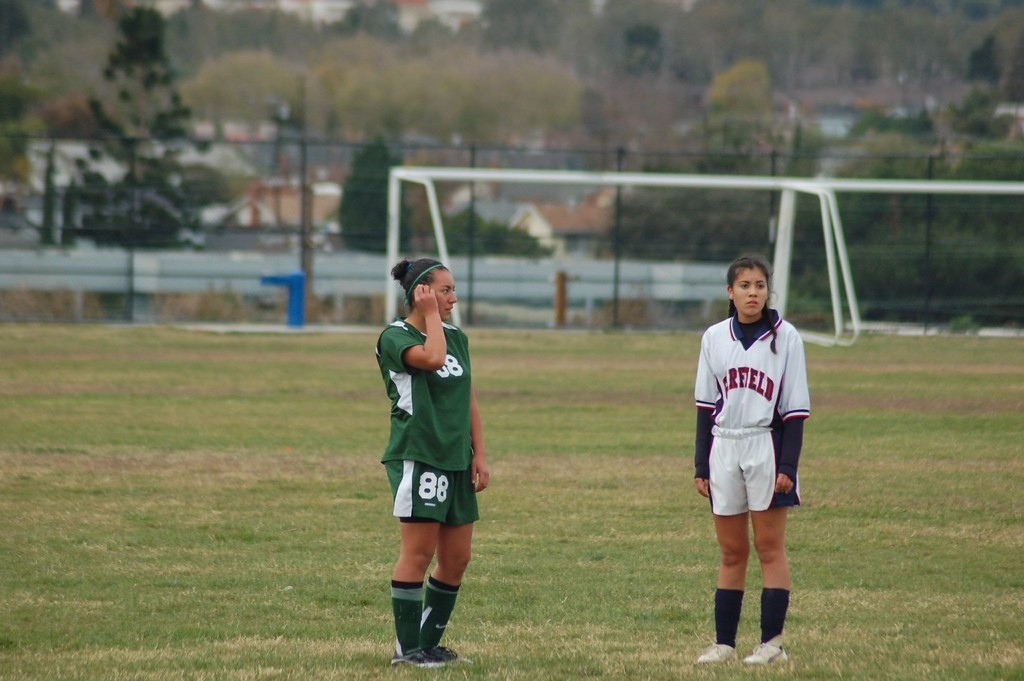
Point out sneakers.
[390,649,446,668]
[696,642,737,665]
[743,644,787,665]
[420,645,475,667]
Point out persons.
[694,256,810,665]
[376,258,489,670]
